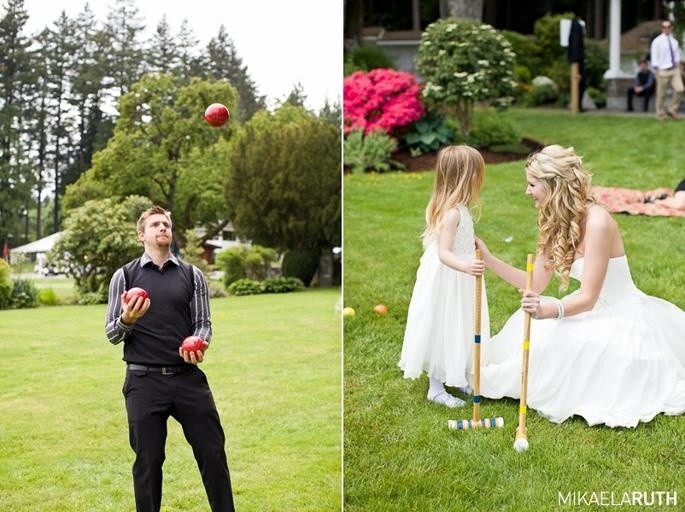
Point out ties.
[668,35,676,68]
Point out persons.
[644,180,685,209]
[627,61,655,113]
[399,144,491,408]
[475,144,684,429]
[104,206,235,512]
[650,20,685,119]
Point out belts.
[128,365,183,376]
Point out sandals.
[427,390,466,408]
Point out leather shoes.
[657,109,679,122]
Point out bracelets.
[555,300,565,320]
